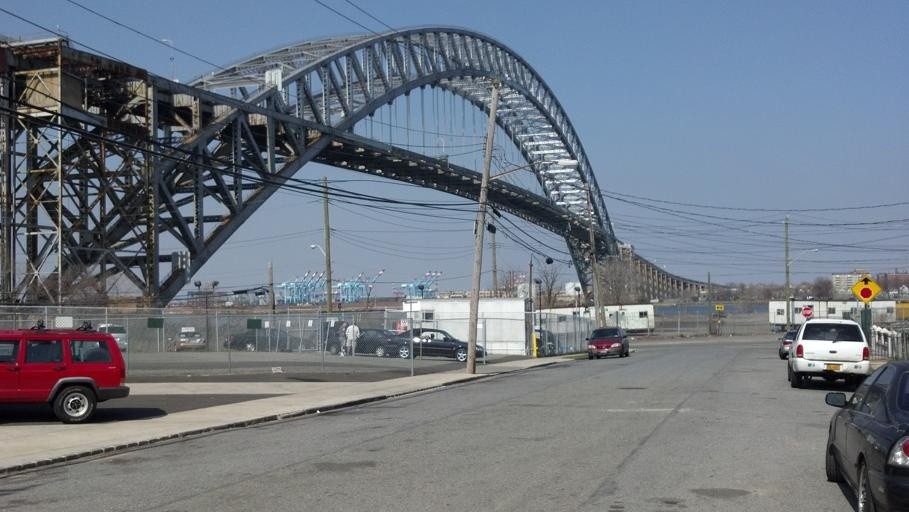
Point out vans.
[97,323,128,351]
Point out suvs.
[0,320,129,424]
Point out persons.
[337,322,348,355]
[344,323,361,356]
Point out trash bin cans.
[710,312,720,333]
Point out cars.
[778,330,798,359]
[825,360,909,512]
[388,328,487,362]
[788,317,870,387]
[586,327,629,357]
[166,331,205,351]
[224,327,313,351]
[534,329,560,357]
[325,328,395,357]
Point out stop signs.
[801,308,812,317]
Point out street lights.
[782,248,818,329]
[465,158,580,372]
[213,281,220,304]
[194,281,201,293]
[418,285,423,360]
[533,277,548,327]
[310,244,333,312]
